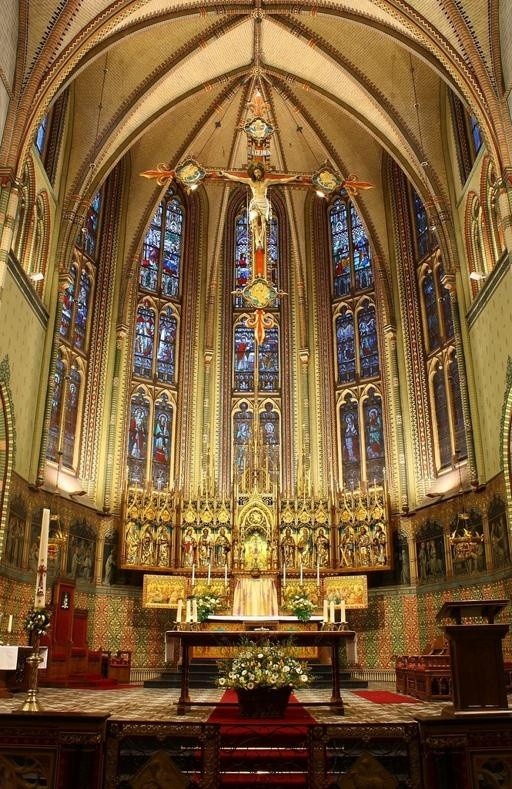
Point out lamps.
[425,492,446,498]
[69,490,87,496]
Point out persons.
[125,510,387,566]
[398,517,507,588]
[133,405,379,465]
[8,514,116,586]
[217,164,299,253]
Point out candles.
[176,563,227,623]
[317,563,319,586]
[300,564,302,585]
[283,563,285,586]
[323,599,346,623]
[7,614,13,633]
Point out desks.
[0,645,48,698]
[166,630,355,716]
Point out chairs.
[390,636,454,702]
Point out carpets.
[349,689,424,704]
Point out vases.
[234,685,292,719]
[18,630,46,712]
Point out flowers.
[187,592,225,622]
[285,592,316,623]
[207,631,317,692]
[22,608,54,636]
